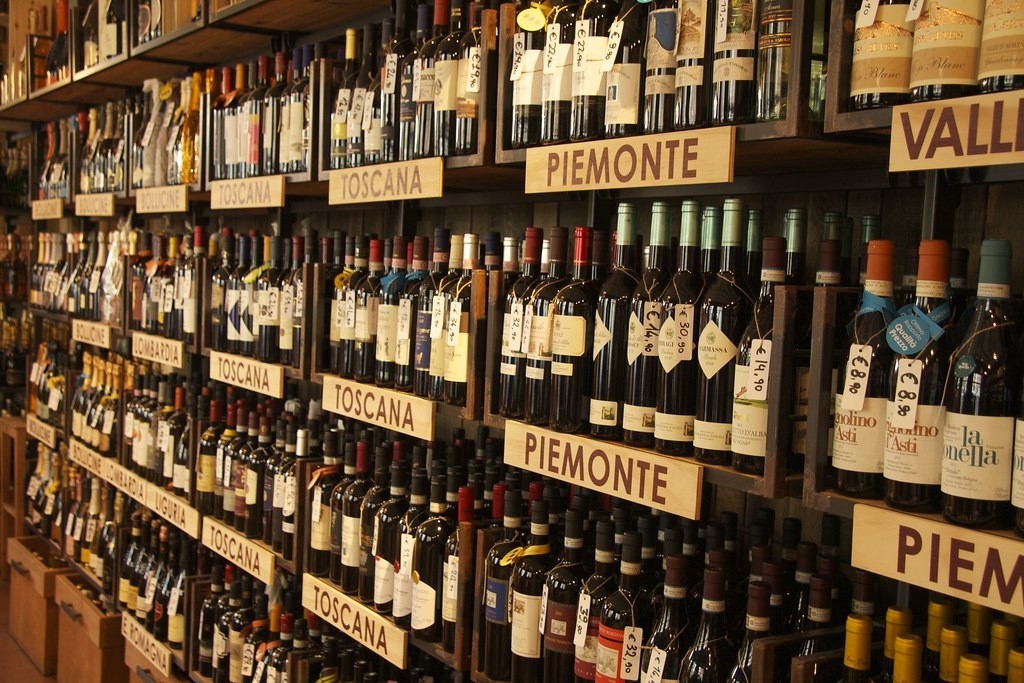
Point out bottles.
[0,0,1024,683]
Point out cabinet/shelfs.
[0,0,1023,683]
